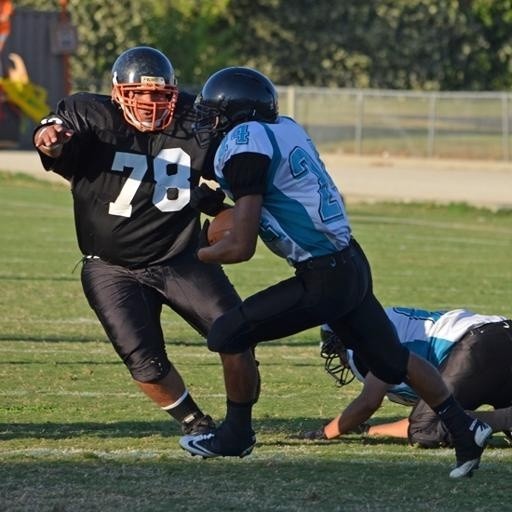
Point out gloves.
[341,423,371,437]
[190,181,226,217]
[192,218,210,265]
[300,424,327,440]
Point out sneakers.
[179,421,256,459]
[449,418,493,479]
[180,412,217,437]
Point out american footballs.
[208,210,234,246]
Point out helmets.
[319,321,357,387]
[195,66,280,126]
[110,44,176,103]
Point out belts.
[72,253,100,273]
[468,318,511,335]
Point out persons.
[321,307,511,448]
[34,46,242,435]
[179,67,494,478]
